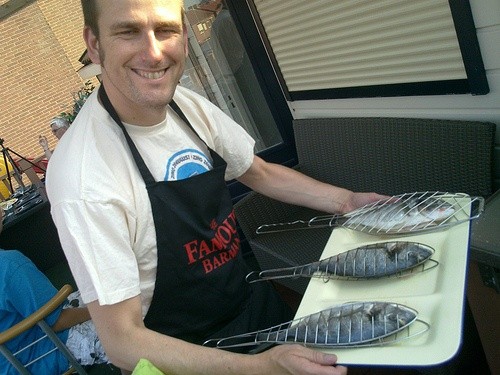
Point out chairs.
[0,284,88,375]
[233,119,496,295]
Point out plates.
[290,193,472,366]
[13,184,42,214]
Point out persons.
[39,0,491,375]
[0,203,120,375]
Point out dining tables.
[0,179,49,231]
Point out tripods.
[0,139,46,196]
